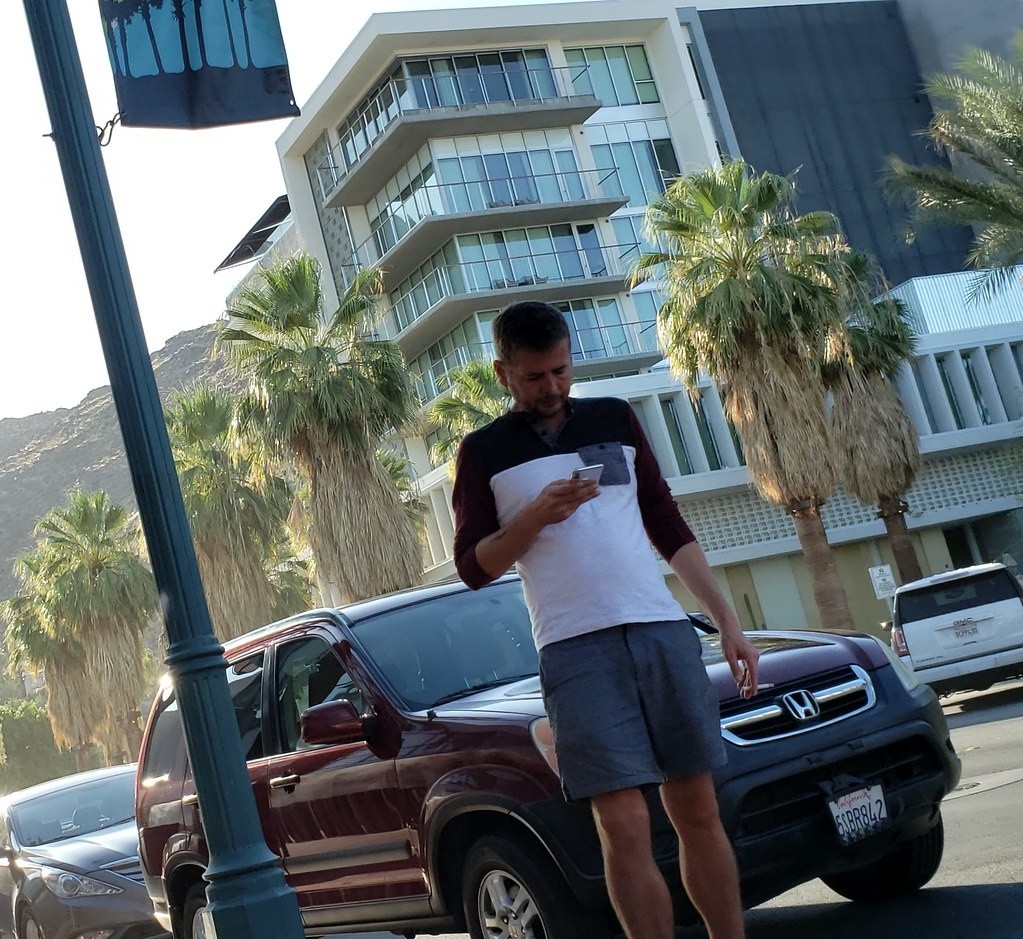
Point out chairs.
[523,273,549,285]
[307,642,423,713]
[71,806,103,830]
[449,623,511,689]
[487,197,540,208]
[493,278,521,287]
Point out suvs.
[133,573,964,939]
[881,561,1023,700]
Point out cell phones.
[571,463,604,490]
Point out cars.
[0,762,174,939]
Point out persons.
[452,301,759,939]
